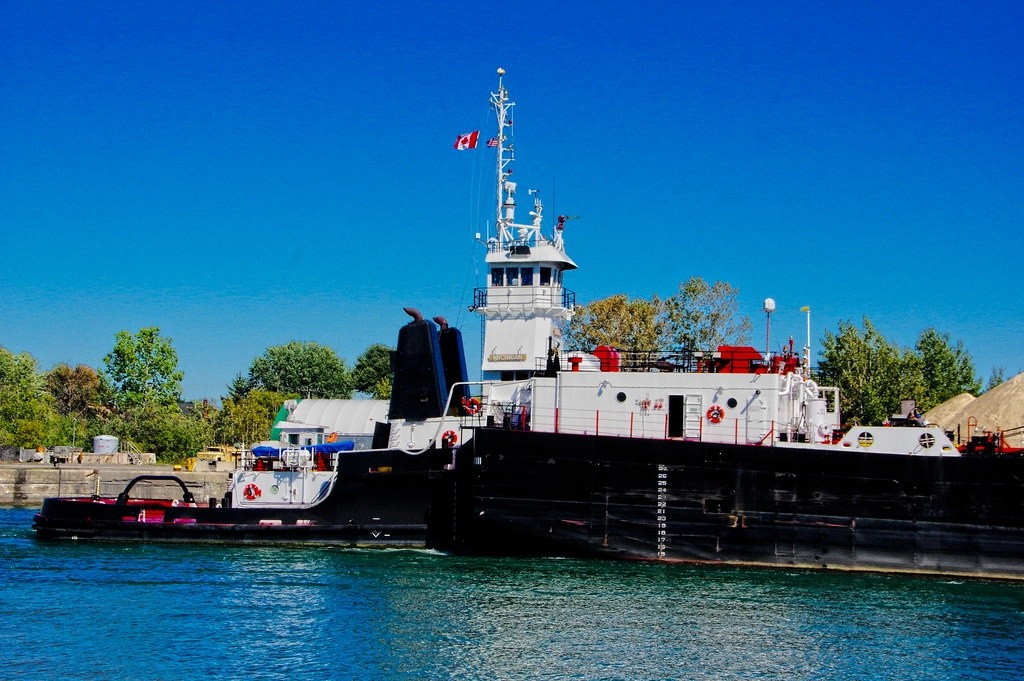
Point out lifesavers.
[707,406,724,423]
[442,430,457,446]
[465,398,481,414]
[243,484,259,500]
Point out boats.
[31,68,1023,581]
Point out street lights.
[801,306,810,375]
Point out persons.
[905,406,922,427]
[77,454,83,463]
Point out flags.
[487,138,498,147]
[454,131,478,150]
